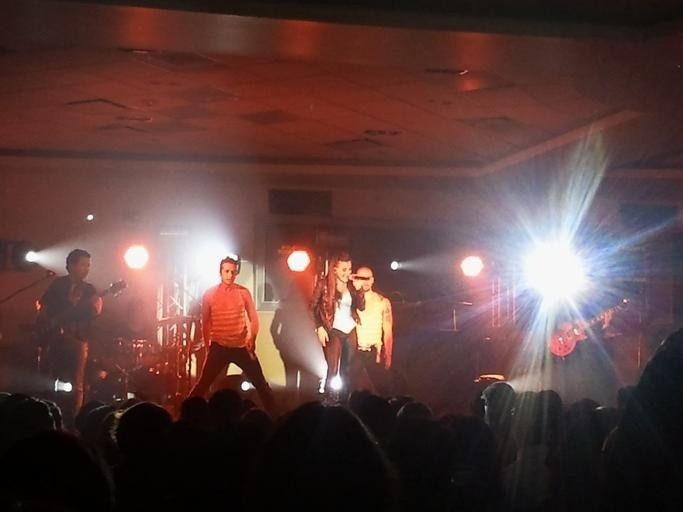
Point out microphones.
[348,275,370,282]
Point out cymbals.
[157,316,201,327]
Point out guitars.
[32,278,129,354]
[544,296,630,357]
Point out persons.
[31,248,104,432]
[354,267,393,396]
[0,327,681,510]
[184,255,280,415]
[302,252,366,403]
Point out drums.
[112,336,153,377]
